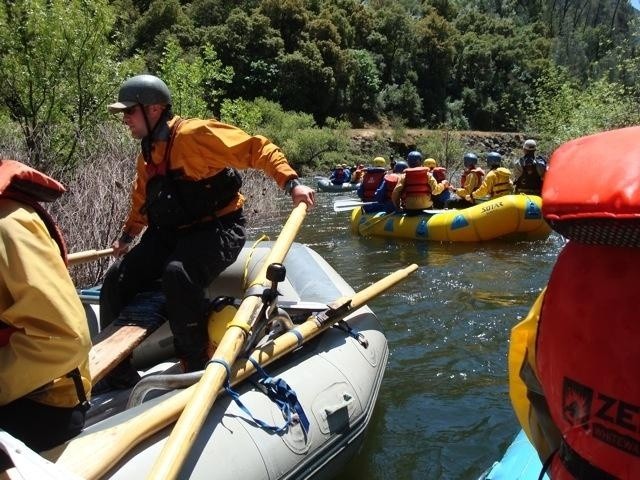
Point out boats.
[479,425,552,480]
[317,178,361,192]
[352,194,553,243]
[1,234,393,477]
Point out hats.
[523,140,537,150]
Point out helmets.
[336,164,364,169]
[407,151,422,167]
[464,153,477,167]
[423,158,436,168]
[107,75,174,116]
[487,152,501,169]
[373,157,386,167]
[393,162,408,173]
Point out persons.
[0,159,93,473]
[507,127,640,480]
[329,140,545,216]
[91,73,317,399]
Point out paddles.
[1,430,85,480]
[423,210,456,213]
[334,201,378,211]
[359,212,396,236]
[2,264,420,479]
